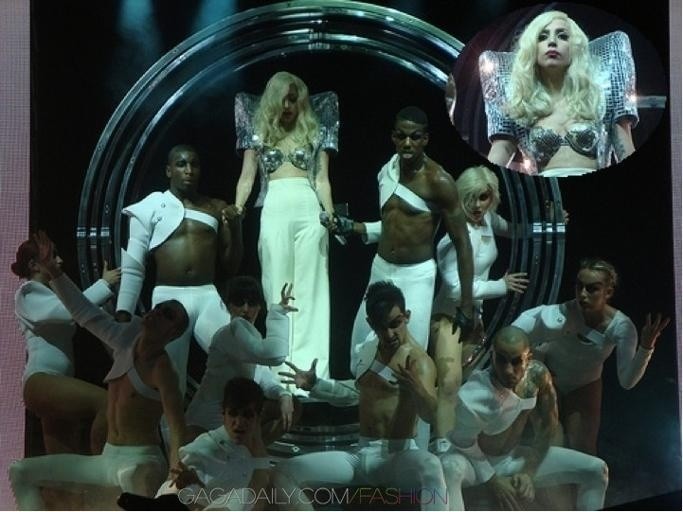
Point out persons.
[325,105,474,374]
[271,280,448,510]
[510,258,672,508]
[8,239,121,457]
[185,277,298,439]
[166,378,313,511]
[476,12,637,178]
[114,141,242,387]
[6,231,182,510]
[431,167,578,431]
[439,325,607,510]
[221,70,343,395]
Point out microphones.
[320,212,349,245]
